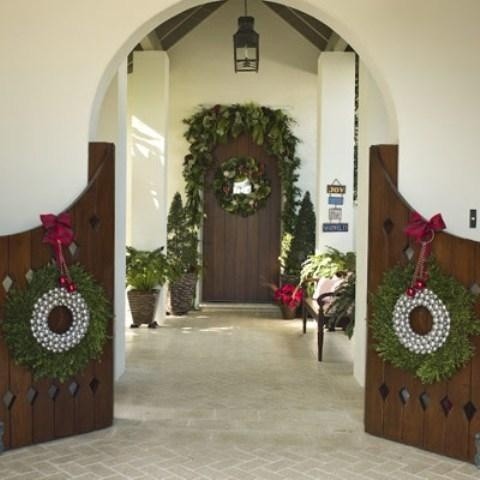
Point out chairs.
[300,270,354,361]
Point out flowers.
[273,284,303,308]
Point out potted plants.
[276,191,315,318]
[166,192,207,315]
[125,245,168,327]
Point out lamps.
[233,1,259,73]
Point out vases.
[279,303,297,318]
